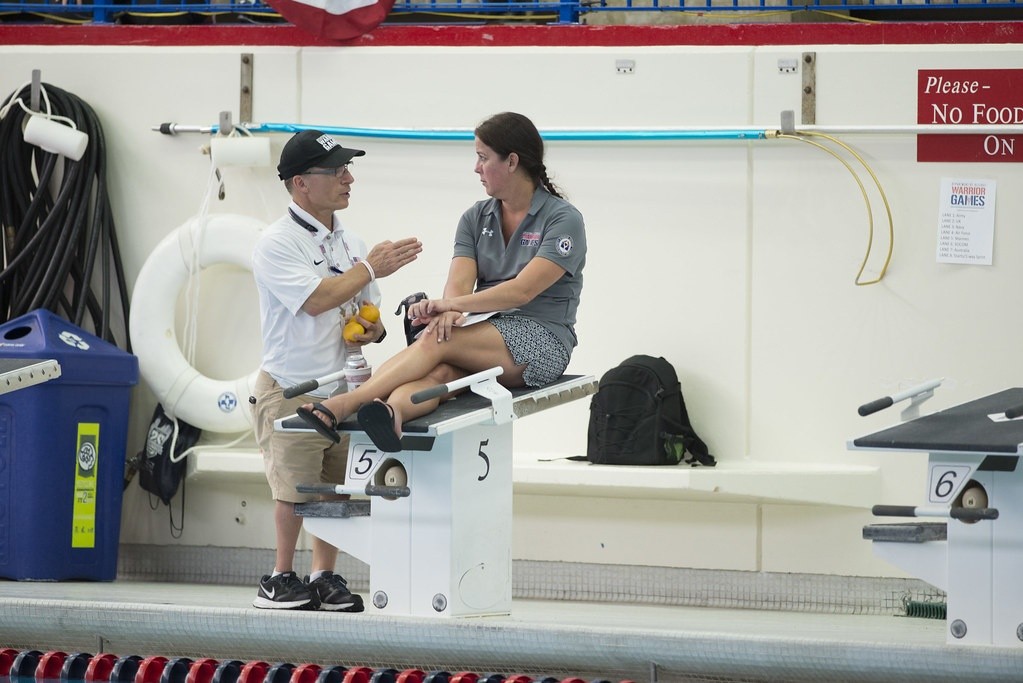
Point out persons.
[253,130,423,612]
[297,112,587,453]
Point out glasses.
[300,161,355,177]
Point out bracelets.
[362,260,375,281]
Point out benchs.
[188,448,878,507]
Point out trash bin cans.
[1,308,140,586]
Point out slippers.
[357,401,402,453]
[296,402,341,443]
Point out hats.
[277,130,366,180]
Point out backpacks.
[137,403,202,539]
[567,354,718,466]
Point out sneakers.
[252,571,319,610]
[303,570,365,612]
